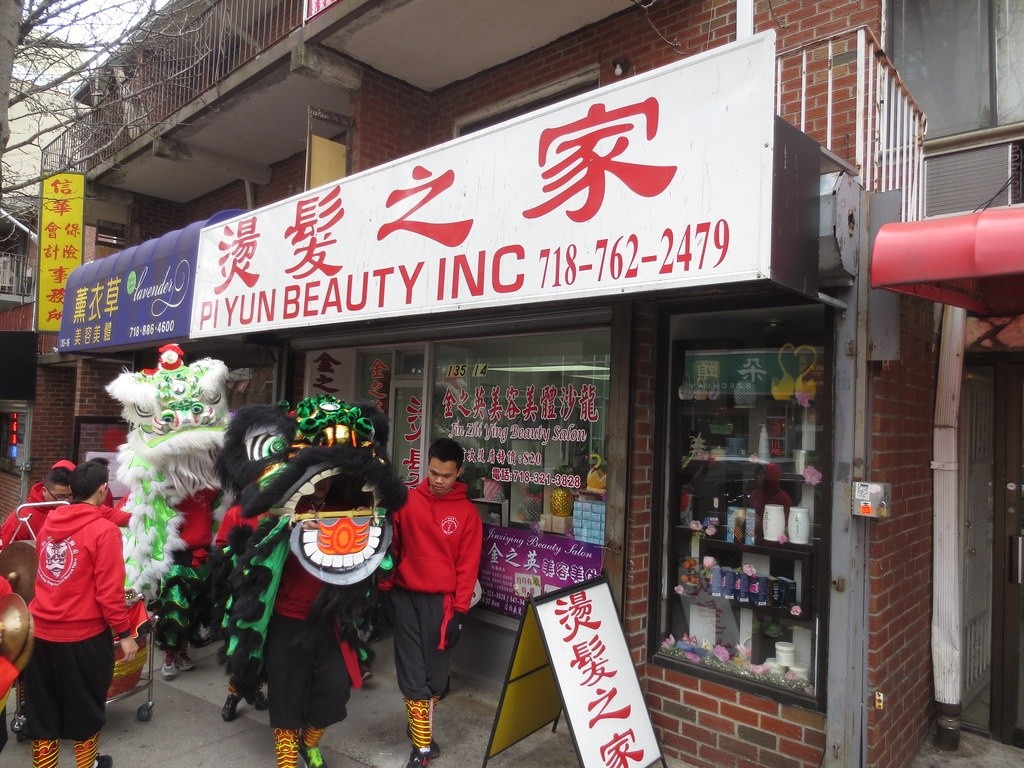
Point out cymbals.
[0,593,34,673]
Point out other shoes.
[369,630,380,642]
[360,662,373,682]
[191,624,215,646]
[95,753,113,768]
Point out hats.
[52,460,76,473]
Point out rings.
[133,654,135,656]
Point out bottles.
[766,642,809,681]
[787,505,810,544]
[763,503,785,542]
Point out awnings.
[57,208,252,352]
[869,207,1024,315]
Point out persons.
[266,473,369,768]
[750,463,792,544]
[378,436,483,768]
[23,463,140,768]
[214,500,266,721]
[0,456,133,753]
[158,488,218,680]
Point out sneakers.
[222,691,243,721]
[160,654,178,681]
[154,638,166,651]
[245,690,267,711]
[297,736,327,768]
[176,652,196,671]
[406,721,440,759]
[406,749,430,768]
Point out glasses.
[44,486,73,502]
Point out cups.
[726,438,748,455]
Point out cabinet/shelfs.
[668,463,820,625]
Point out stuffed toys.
[107,342,407,709]
